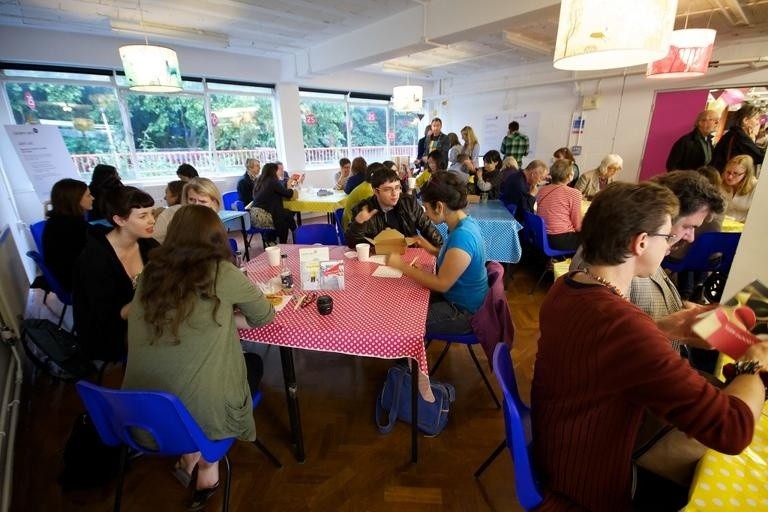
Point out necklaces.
[582,267,631,302]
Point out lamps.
[643,1,716,80]
[118,0,183,92]
[551,1,678,72]
[392,55,423,112]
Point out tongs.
[302,291,317,308]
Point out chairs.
[421,262,504,411]
[507,203,516,216]
[74,378,282,512]
[28,220,51,256]
[222,191,239,210]
[472,341,547,512]
[25,249,74,330]
[522,211,576,295]
[291,223,340,245]
[660,232,740,280]
[336,206,350,244]
[235,201,278,260]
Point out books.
[391,156,411,174]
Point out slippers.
[186,479,220,511]
[170,457,190,488]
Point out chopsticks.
[294,294,306,310]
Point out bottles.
[236,251,247,276]
[281,254,294,295]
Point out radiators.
[0,224,31,343]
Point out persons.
[446,132,462,166]
[534,159,585,250]
[237,159,261,205]
[252,164,297,245]
[696,166,723,187]
[346,156,371,192]
[384,159,413,195]
[417,124,432,162]
[460,124,479,167]
[667,109,721,170]
[498,156,518,183]
[713,106,764,167]
[342,162,384,230]
[573,153,623,201]
[501,120,530,169]
[718,154,757,223]
[553,148,578,186]
[345,170,445,251]
[448,154,471,187]
[501,160,548,220]
[154,177,222,246]
[414,150,446,189]
[86,164,127,219]
[276,160,290,189]
[175,163,200,182]
[334,158,349,188]
[567,170,768,350]
[387,172,492,367]
[75,187,163,373]
[526,181,768,512]
[466,150,503,204]
[124,204,276,506]
[164,181,189,206]
[422,118,448,169]
[42,178,115,300]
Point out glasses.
[648,233,676,241]
[728,171,745,176]
[376,185,400,191]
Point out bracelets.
[721,359,768,401]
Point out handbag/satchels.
[19,319,97,380]
[376,361,456,439]
[250,207,274,230]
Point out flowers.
[395,163,414,184]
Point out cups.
[356,243,370,262]
[480,193,488,204]
[265,246,280,266]
